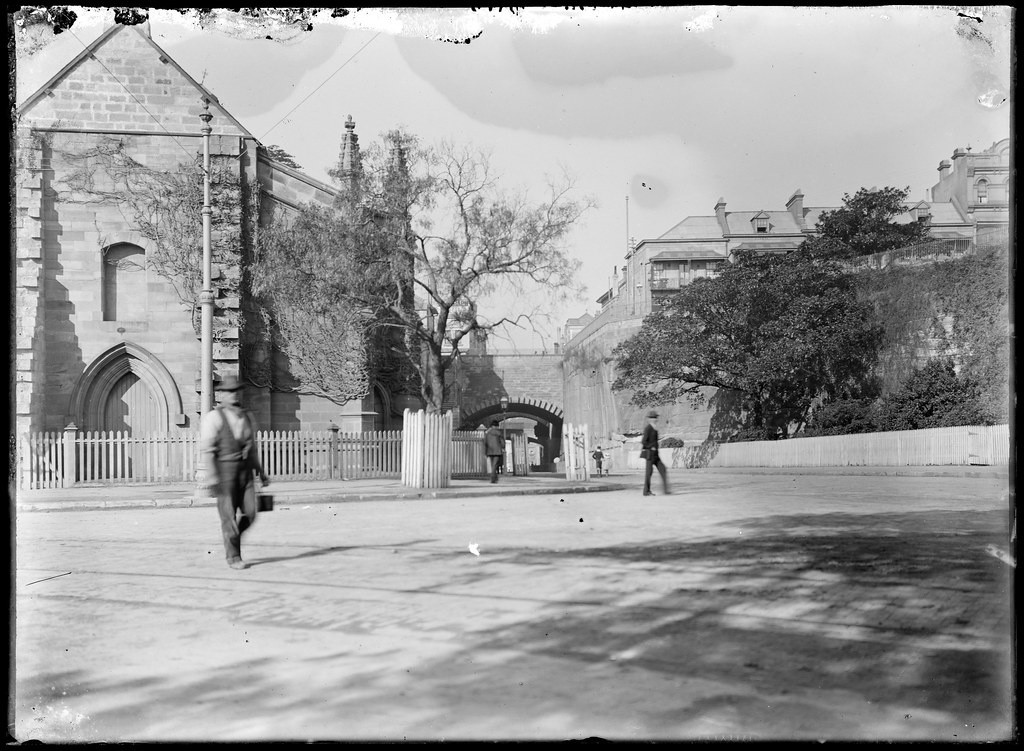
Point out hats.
[214,374,250,392]
[490,420,499,426]
[645,411,660,417]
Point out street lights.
[500,395,508,477]
[196,94,213,498]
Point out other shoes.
[643,493,656,496]
[230,561,247,569]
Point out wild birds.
[468,541,480,556]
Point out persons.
[638,410,672,496]
[603,453,613,477]
[592,447,604,477]
[200,374,271,570]
[484,419,506,483]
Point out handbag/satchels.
[257,485,274,512]
[640,448,649,460]
